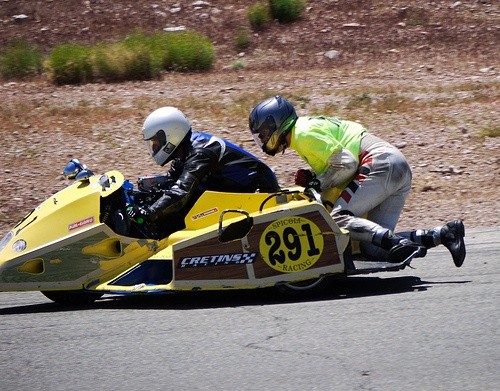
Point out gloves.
[294,169,313,187]
[304,178,321,198]
[128,205,150,219]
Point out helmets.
[140,106,192,166]
[248,95,299,156]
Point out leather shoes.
[439,219,466,267]
[387,230,426,263]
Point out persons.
[128,106,282,225]
[249,96,466,268]
